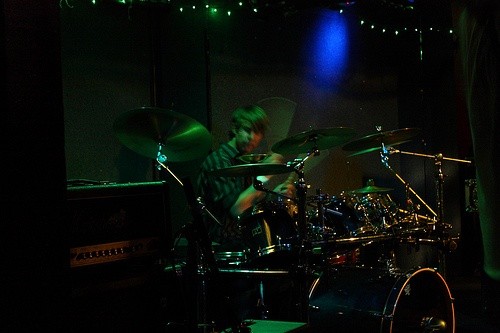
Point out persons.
[197,104,289,250]
[459,0,500,282]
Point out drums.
[255,244,300,274]
[308,266,455,333]
[353,193,399,226]
[213,250,252,271]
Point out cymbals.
[207,162,296,177]
[236,153,266,162]
[342,128,419,152]
[347,186,394,194]
[111,106,211,163]
[345,140,412,159]
[271,127,357,155]
